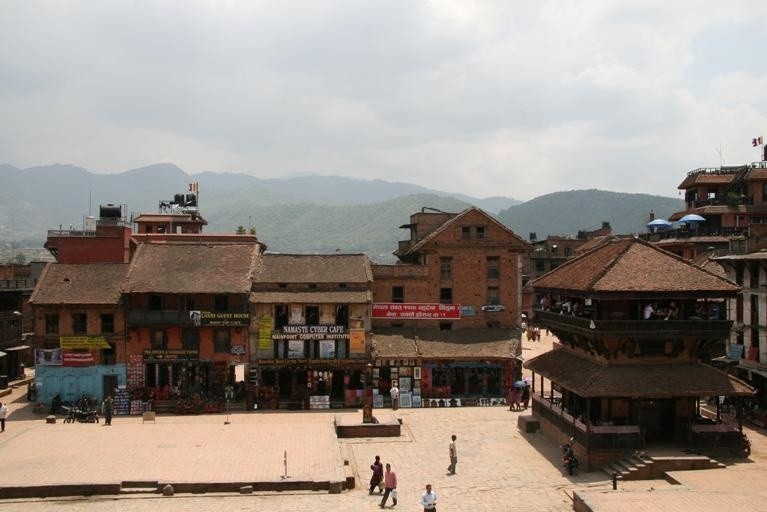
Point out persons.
[421,485,437,512]
[0,402,8,431]
[368,456,383,495]
[447,434,457,474]
[390,384,399,410]
[563,444,572,456]
[509,381,530,410]
[561,448,568,462]
[102,394,114,424]
[378,464,397,508]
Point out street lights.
[14,311,38,367]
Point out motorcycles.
[62,401,101,425]
[559,435,582,477]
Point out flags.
[188,182,198,192]
[751,136,763,147]
